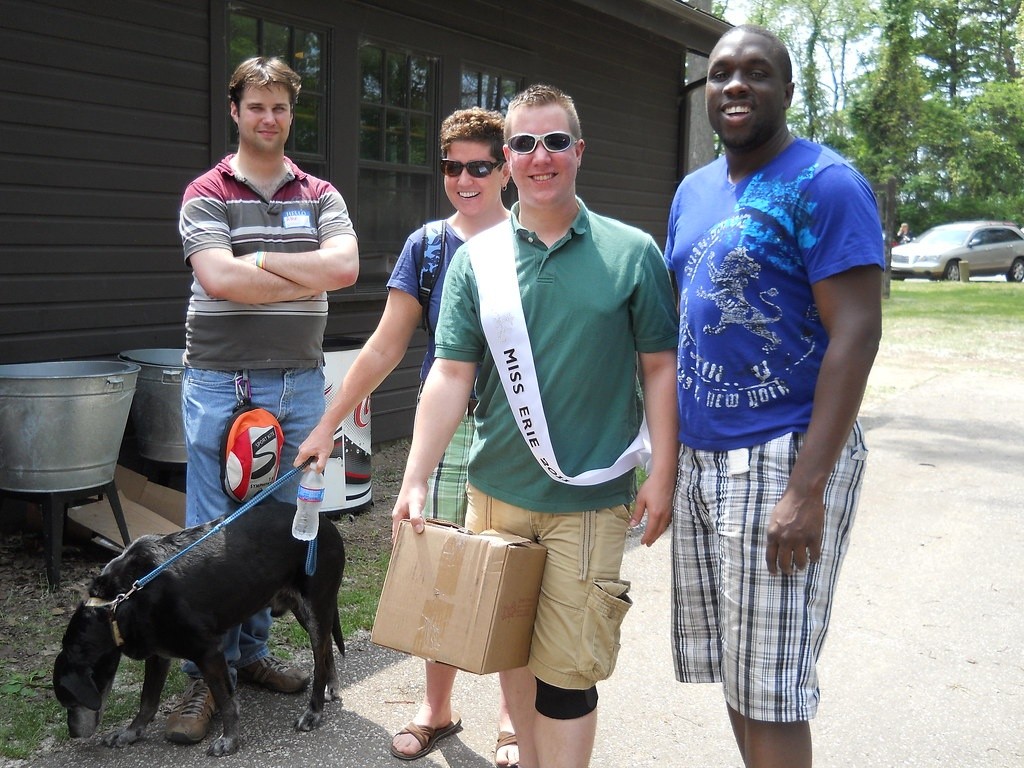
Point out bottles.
[291,462,326,540]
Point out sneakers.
[232,652,309,694]
[163,676,222,745]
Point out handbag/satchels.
[217,390,286,509]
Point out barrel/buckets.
[118,349,187,463]
[319,335,373,520]
[0,359,141,492]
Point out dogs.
[52,504,347,757]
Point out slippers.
[495,719,520,768]
[388,710,462,761]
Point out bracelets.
[255,250,267,270]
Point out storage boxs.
[370,516,547,676]
[67,464,186,554]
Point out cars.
[891,221,1024,281]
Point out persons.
[897,222,913,244]
[391,84,680,768]
[664,23,887,768]
[162,54,359,745]
[292,104,520,768]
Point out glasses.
[505,133,578,156]
[439,158,506,179]
